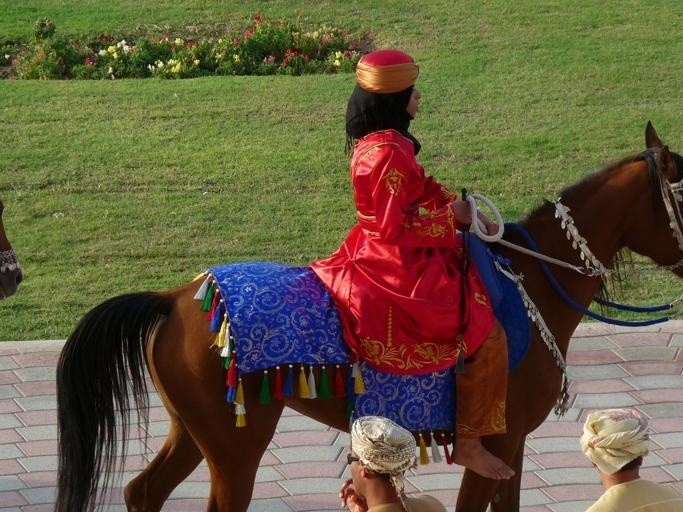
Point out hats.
[578,406,652,475]
[350,414,416,475]
[354,49,420,94]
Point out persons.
[305,45,519,483]
[336,413,448,511]
[577,405,682,512]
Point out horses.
[0,198,25,303]
[53,118,683,512]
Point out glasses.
[345,450,359,464]
